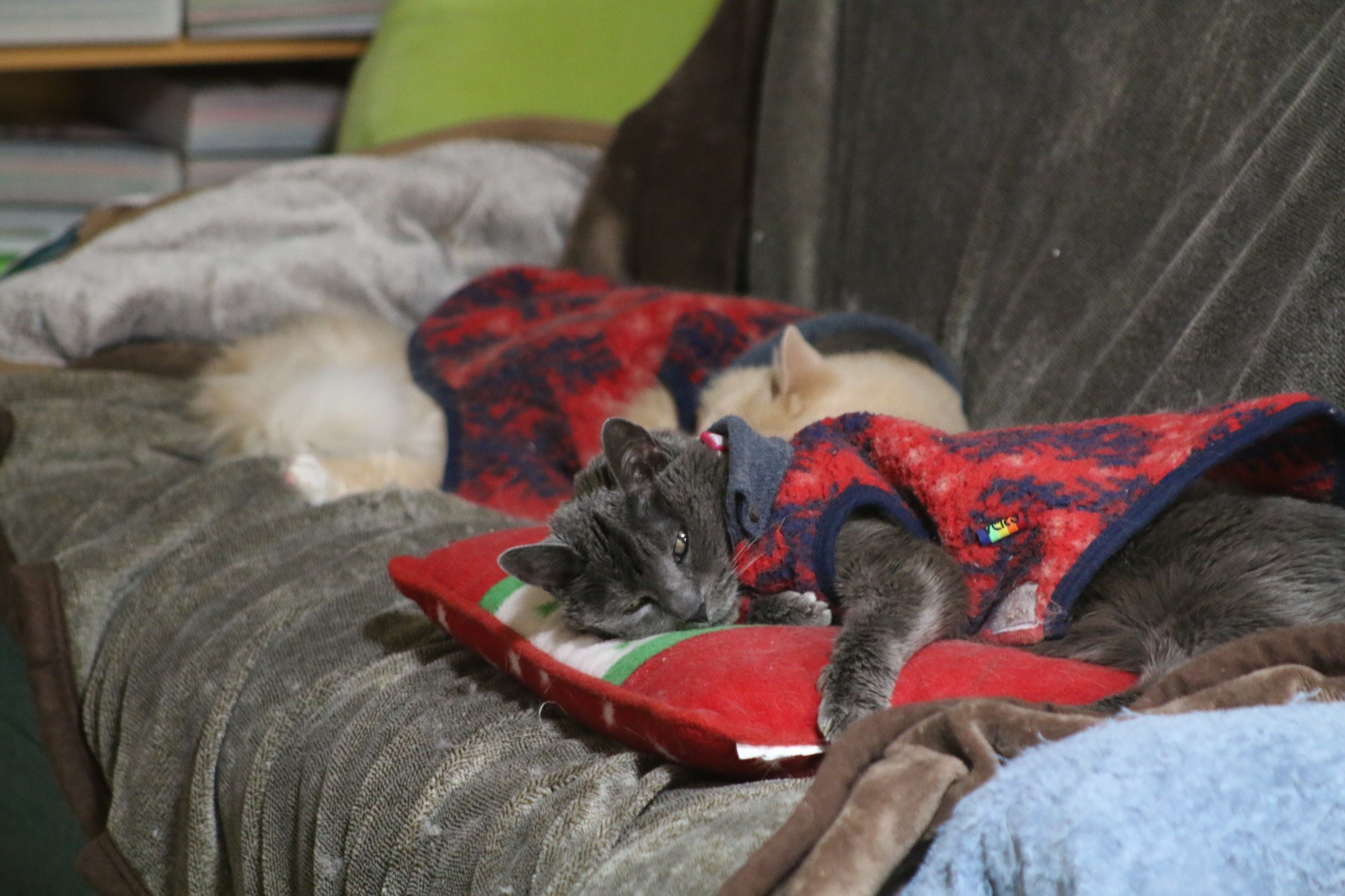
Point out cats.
[201,254,972,497]
[486,408,1345,752]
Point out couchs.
[0,0,1345,896]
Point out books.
[0,0,381,46]
[0,74,344,214]
